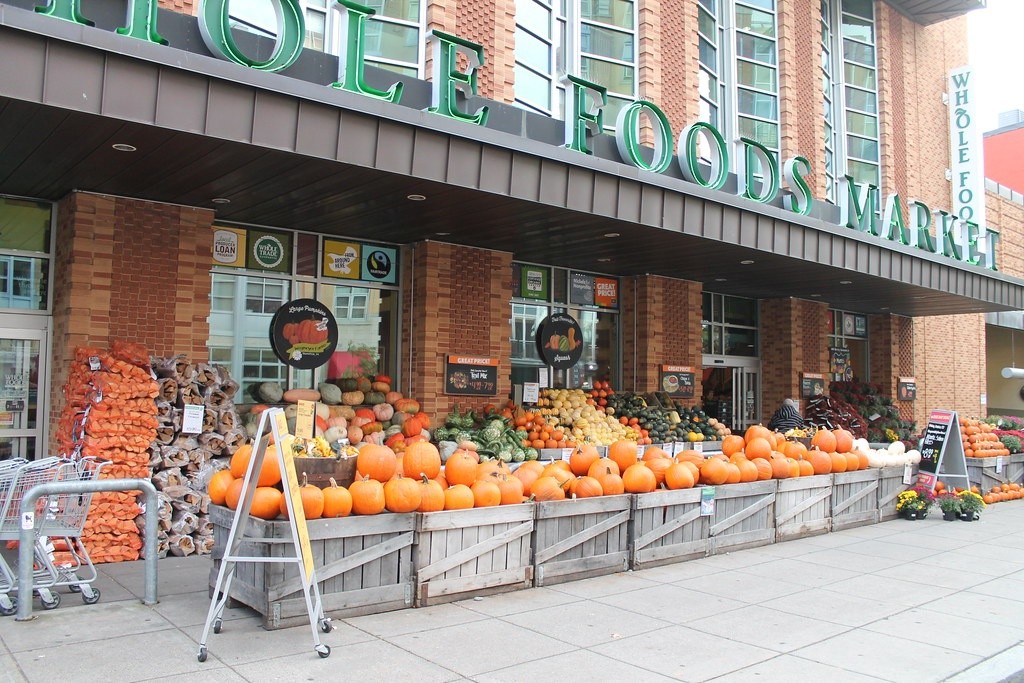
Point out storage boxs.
[832,467,879,532]
[877,463,918,524]
[414,495,534,608]
[536,446,609,466]
[208,501,415,631]
[707,478,778,558]
[966,456,1011,498]
[1010,453,1024,487]
[775,473,834,543]
[673,440,723,461]
[631,484,709,571]
[534,491,632,588]
[608,443,673,461]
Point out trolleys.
[0,455,113,615]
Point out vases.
[942,510,955,520]
[917,508,927,520]
[906,508,918,521]
[960,510,974,521]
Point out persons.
[767,398,805,434]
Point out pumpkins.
[211,373,1024,517]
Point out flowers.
[909,488,936,506]
[938,492,963,516]
[896,490,926,511]
[957,490,986,513]
